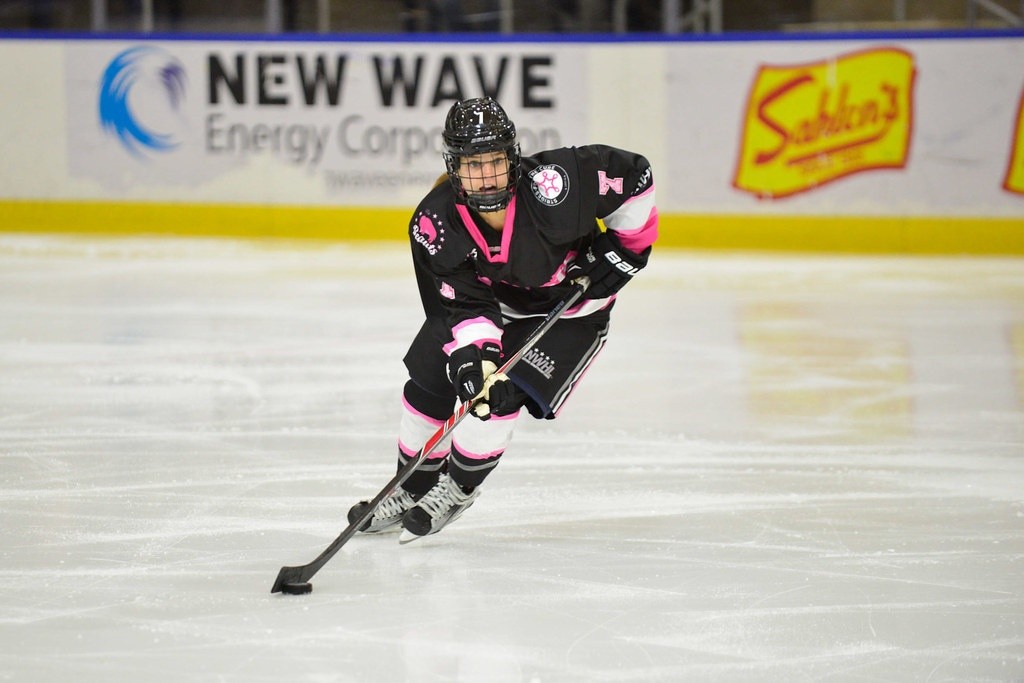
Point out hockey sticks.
[271,274,591,595]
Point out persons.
[349,97,656,534]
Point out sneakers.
[347,490,416,538]
[401,472,479,544]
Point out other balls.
[282,583,313,595]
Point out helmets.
[441,98,522,210]
